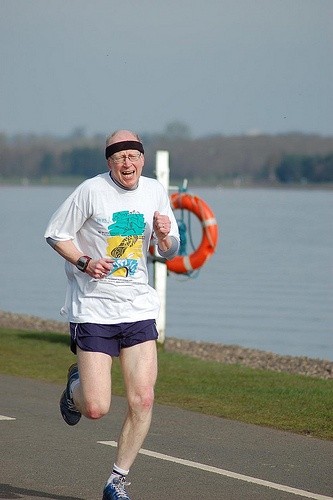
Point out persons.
[44,129,178,500]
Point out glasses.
[109,151,142,162]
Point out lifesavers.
[150,192,219,275]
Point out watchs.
[76,256,91,272]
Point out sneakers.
[59,363,87,426]
[101,475,131,500]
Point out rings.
[163,224,164,227]
